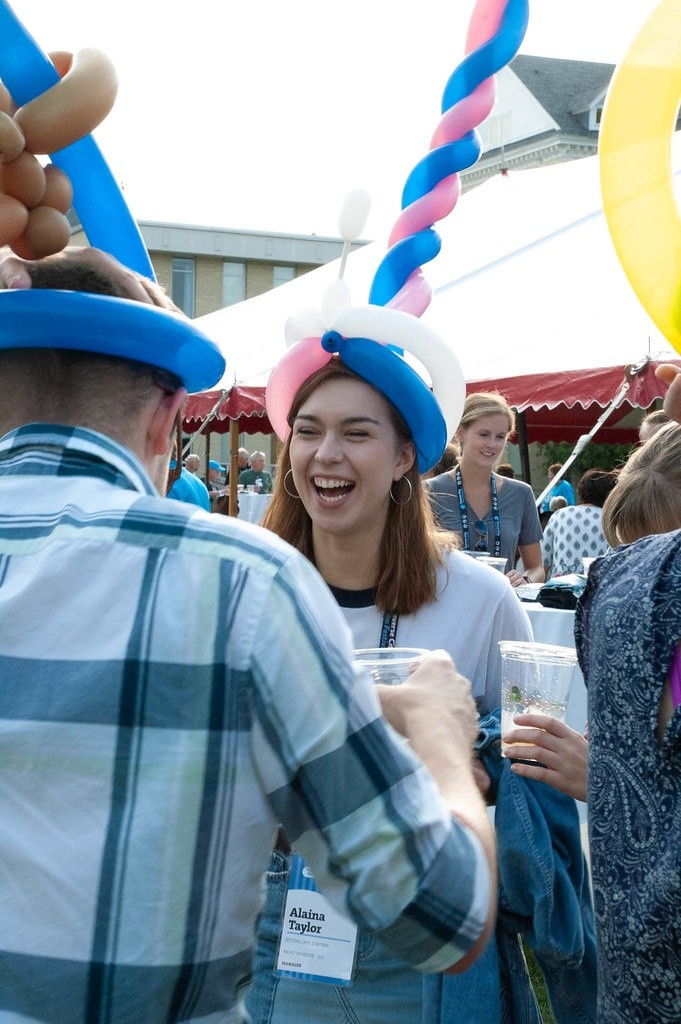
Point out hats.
[209,460,226,472]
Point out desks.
[518,600,589,874]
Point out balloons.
[0,0,226,395]
[367,0,529,318]
[597,0,681,352]
[262,301,467,474]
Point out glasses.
[471,520,489,551]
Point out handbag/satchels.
[537,573,588,610]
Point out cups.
[247,485,254,495]
[497,640,578,762]
[463,551,491,559]
[356,646,432,686]
[237,485,244,491]
[582,557,597,576]
[479,556,508,574]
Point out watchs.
[522,575,532,584]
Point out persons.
[496,464,515,480]
[427,443,459,479]
[199,459,229,499]
[236,451,272,493]
[504,358,681,1022]
[214,493,240,518]
[0,264,496,1024]
[424,390,545,591]
[166,440,212,512]
[225,448,252,488]
[184,453,200,475]
